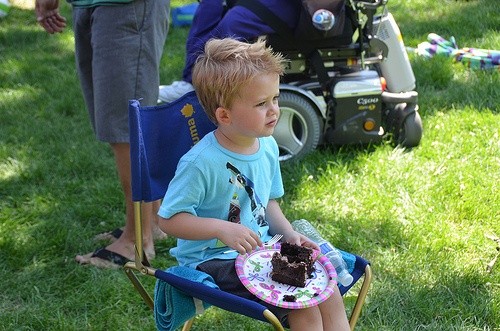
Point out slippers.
[77,247,135,271]
[91,226,126,244]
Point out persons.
[159,0,300,105]
[35,0,171,269]
[157,36,351,331]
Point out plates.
[234,243,338,309]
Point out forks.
[224,234,283,254]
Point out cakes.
[270,242,315,287]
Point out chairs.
[124,90,373,331]
